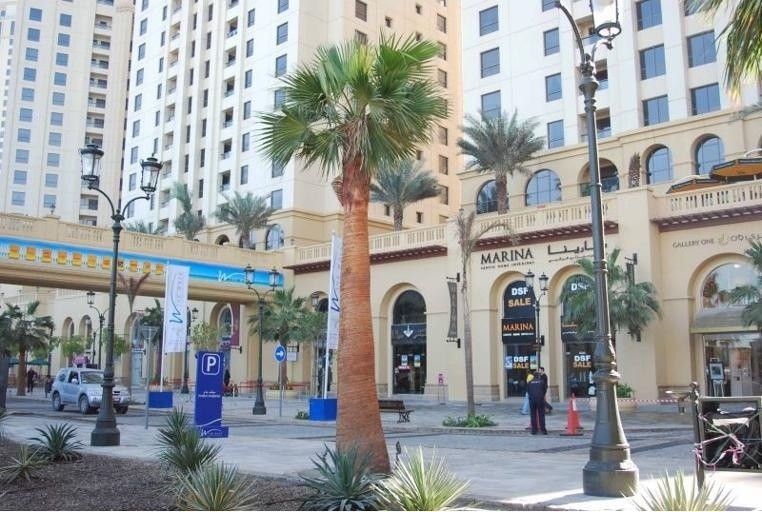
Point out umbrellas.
[9,356,20,365]
[27,358,51,378]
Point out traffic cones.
[559,392,585,437]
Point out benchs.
[378,400,414,423]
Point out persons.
[26,367,38,393]
[521,367,553,416]
[527,371,549,436]
[224,369,231,386]
[71,375,79,384]
[44,375,53,393]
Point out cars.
[50,368,131,413]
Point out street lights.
[547,0,638,499]
[243,262,280,415]
[524,269,549,367]
[78,138,162,447]
